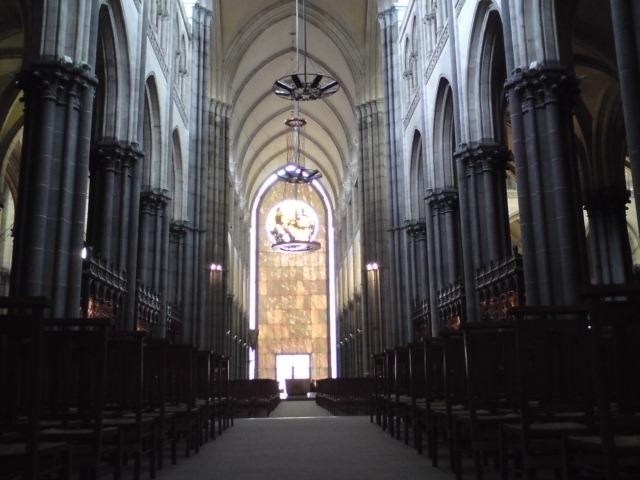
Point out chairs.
[210,377,279,419]
[376,299,639,478]
[2,311,234,478]
[317,377,376,416]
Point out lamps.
[272,0,341,101]
[270,0,323,252]
